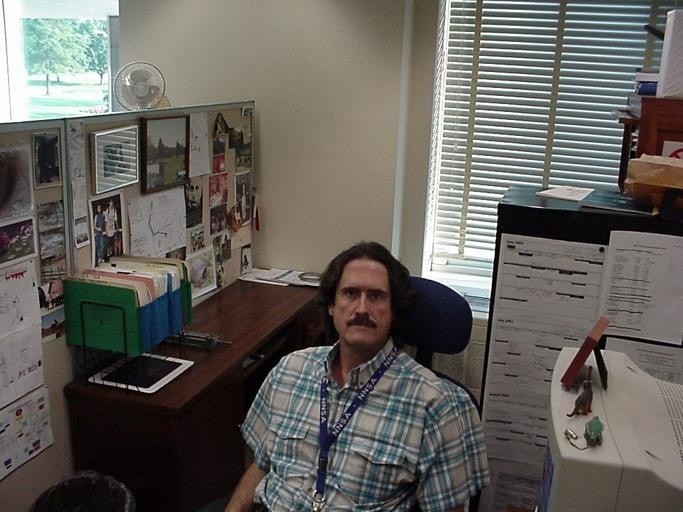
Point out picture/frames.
[560,316,610,391]
[90,123,139,195]
[140,113,190,194]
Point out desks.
[62,266,326,512]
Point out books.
[236,267,322,286]
[624,68,661,162]
[535,182,657,216]
[79,253,187,307]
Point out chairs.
[392,274,482,512]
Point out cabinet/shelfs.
[615,95,683,193]
[480,188,682,511]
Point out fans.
[112,60,172,112]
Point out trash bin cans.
[30,474,134,512]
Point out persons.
[239,182,250,223]
[186,177,201,209]
[93,203,104,263]
[102,198,119,255]
[224,241,492,512]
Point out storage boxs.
[635,82,657,94]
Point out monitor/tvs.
[535,347,683,512]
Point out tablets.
[88,352,194,394]
